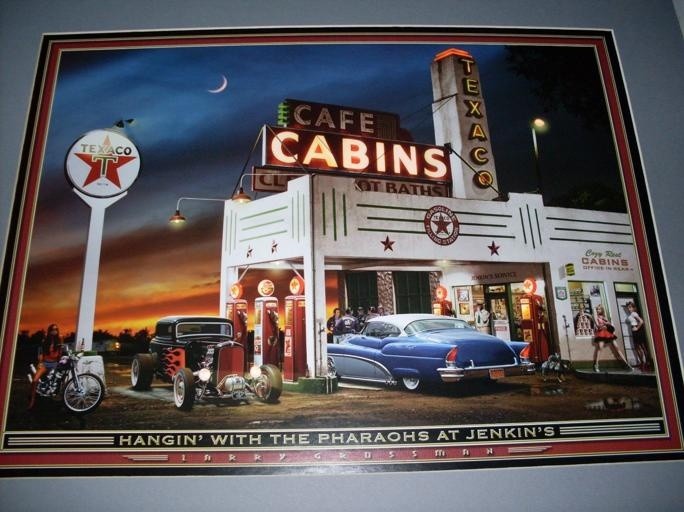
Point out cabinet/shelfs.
[570,291,593,319]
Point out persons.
[327,305,391,344]
[592,304,634,372]
[626,301,647,364]
[475,299,490,334]
[24,324,62,411]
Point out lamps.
[169,197,230,223]
[232,174,307,204]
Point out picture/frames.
[456,288,470,315]
